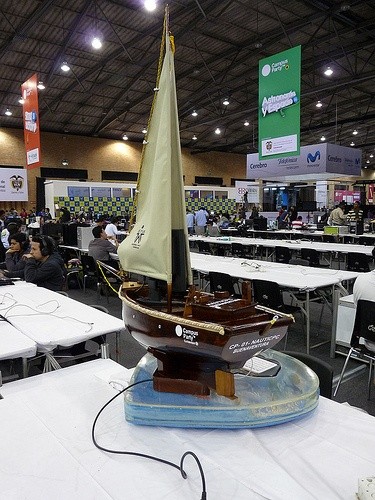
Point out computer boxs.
[76,227,95,249]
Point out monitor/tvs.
[282,191,296,206]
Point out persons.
[249,207,259,218]
[105,220,125,243]
[279,206,298,222]
[330,201,363,226]
[0,207,70,249]
[244,191,249,203]
[0,232,31,271]
[23,237,63,291]
[88,226,118,270]
[186,204,246,237]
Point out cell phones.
[152,347,229,373]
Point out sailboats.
[95,6,296,399]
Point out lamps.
[37,42,46,90]
[192,128,198,141]
[122,131,128,141]
[324,15,334,76]
[5,92,12,117]
[60,59,70,72]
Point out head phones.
[37,235,49,256]
[20,231,30,251]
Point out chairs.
[333,299,375,400]
[57,246,122,304]
[208,272,304,352]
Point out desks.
[190,252,366,355]
[0,279,125,385]
[190,235,375,271]
[221,226,375,244]
